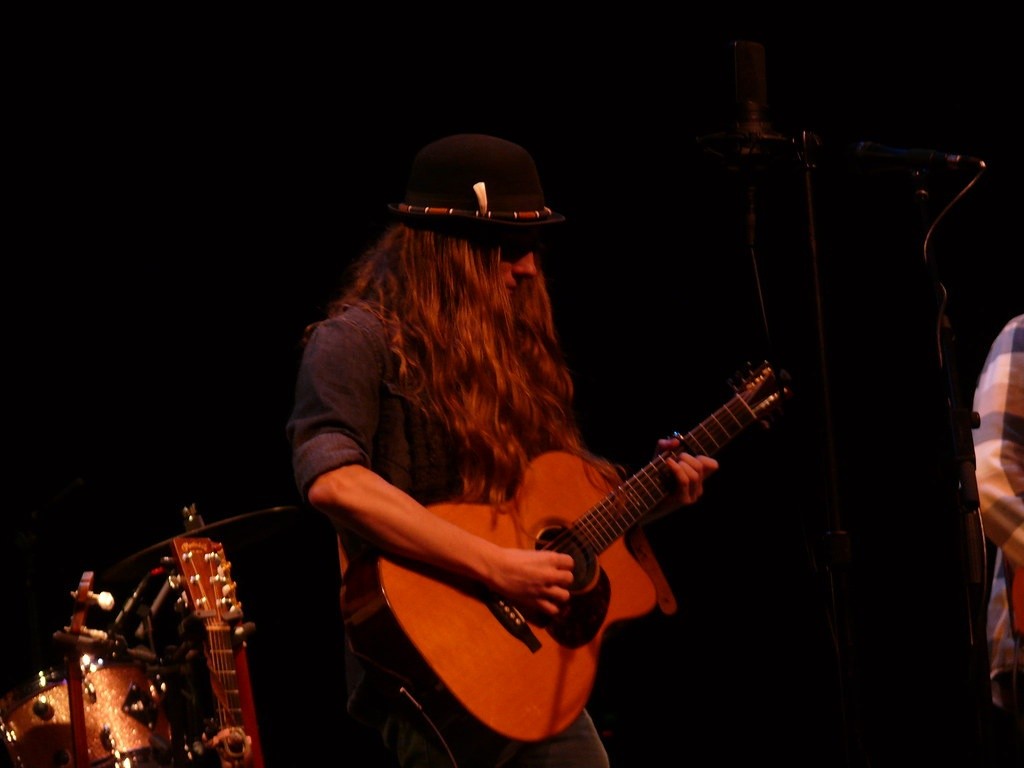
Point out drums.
[0,652,171,767]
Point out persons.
[968,313,1024,710]
[285,134,718,768]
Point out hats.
[388,134,565,227]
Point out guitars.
[343,355,797,744]
[159,535,252,768]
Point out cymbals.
[98,506,300,583]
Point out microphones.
[852,141,987,175]
[108,572,153,636]
[135,570,178,639]
[729,41,772,244]
